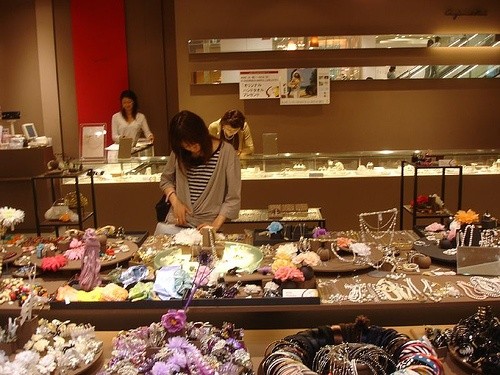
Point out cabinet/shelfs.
[400,160,462,231]
[30,168,98,237]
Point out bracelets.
[257,316,445,375]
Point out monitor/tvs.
[1,111,20,120]
[23,122,38,140]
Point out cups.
[310,37,320,49]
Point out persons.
[111,89,154,143]
[288,70,302,99]
[159,110,241,234]
[208,109,253,158]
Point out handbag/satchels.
[155,190,176,222]
[43,198,69,220]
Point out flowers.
[0,193,482,375]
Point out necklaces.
[224,130,234,140]
[321,207,500,303]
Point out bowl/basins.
[152,241,264,273]
